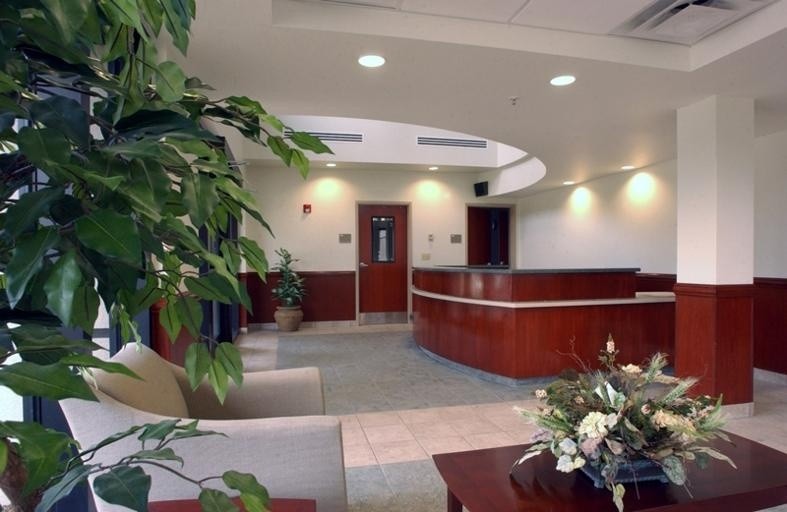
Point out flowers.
[509,331,738,511]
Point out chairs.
[57,341,350,512]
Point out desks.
[431,427,787,512]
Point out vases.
[578,444,670,489]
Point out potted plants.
[269,247,307,331]
